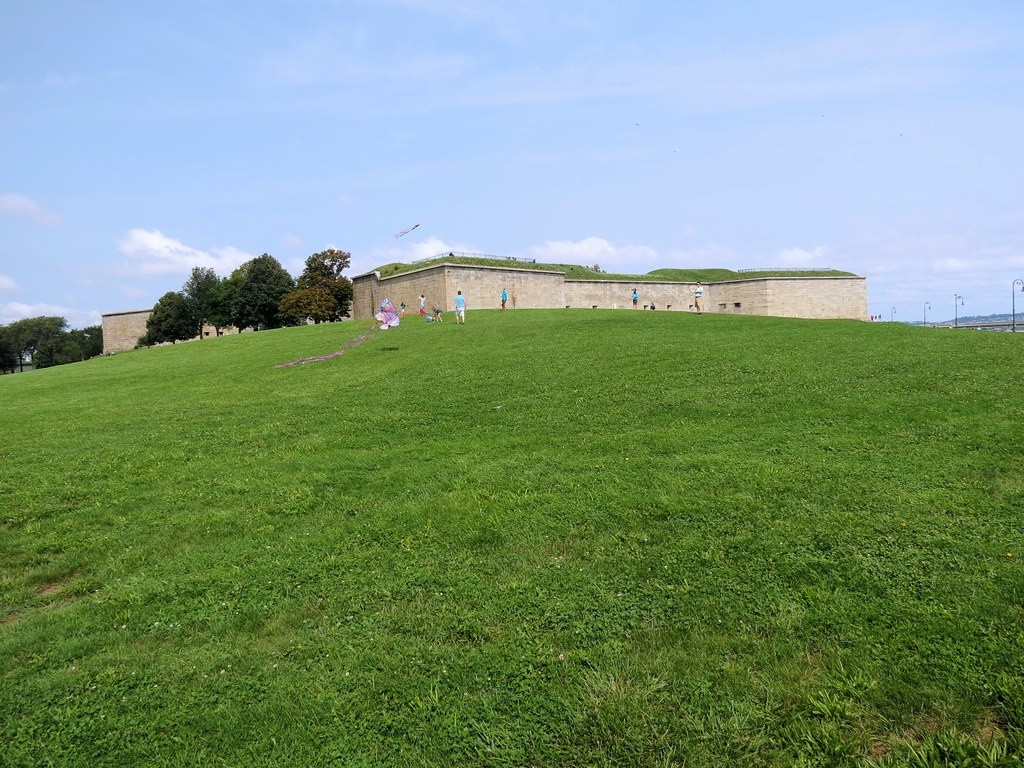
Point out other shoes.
[462,322,465,325]
[697,312,701,315]
[440,319,442,322]
[436,319,438,322]
[457,322,459,325]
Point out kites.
[276,298,400,368]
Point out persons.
[501,288,508,311]
[690,281,703,314]
[870,314,882,322]
[632,288,639,311]
[401,300,406,315]
[454,291,467,324]
[431,306,443,322]
[419,294,426,317]
